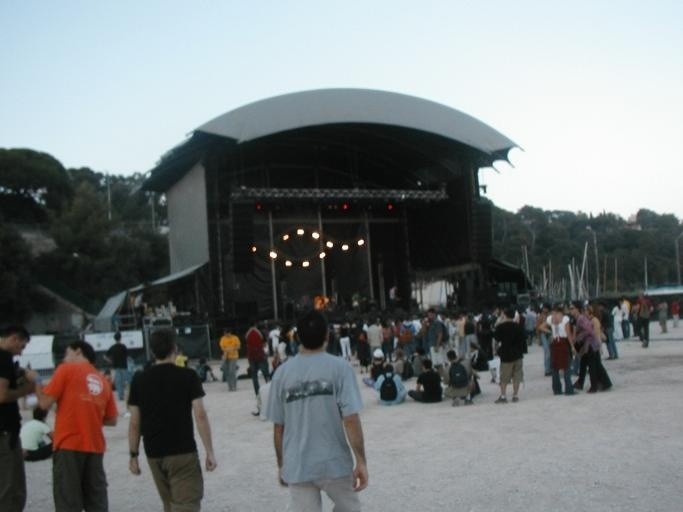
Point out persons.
[101,333,137,401]
[15,361,43,385]
[244,318,301,408]
[672,297,680,330]
[326,287,653,408]
[0,326,26,512]
[264,309,370,512]
[26,340,118,512]
[173,343,218,382]
[657,297,668,334]
[18,408,55,461]
[217,327,240,391]
[126,327,217,512]
[52,316,84,372]
[130,290,146,318]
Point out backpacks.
[402,361,413,380]
[380,375,396,401]
[372,364,383,381]
[442,324,449,341]
[449,361,468,388]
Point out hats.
[374,349,383,358]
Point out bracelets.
[128,451,139,458]
